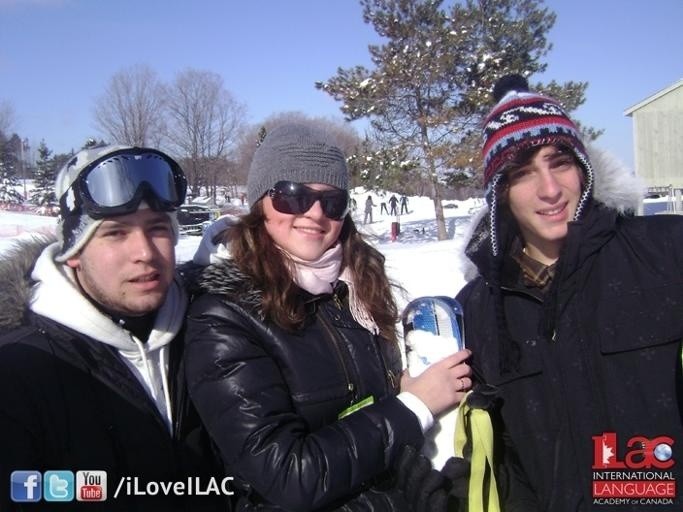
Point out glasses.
[266,181,356,221]
[60,148,187,220]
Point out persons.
[0,140,230,510]
[183,123,475,512]
[362,193,409,225]
[399,74,682,512]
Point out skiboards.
[402,297,466,466]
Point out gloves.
[397,445,470,512]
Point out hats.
[54,145,179,263]
[482,74,594,257]
[248,124,348,210]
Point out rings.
[459,378,465,391]
